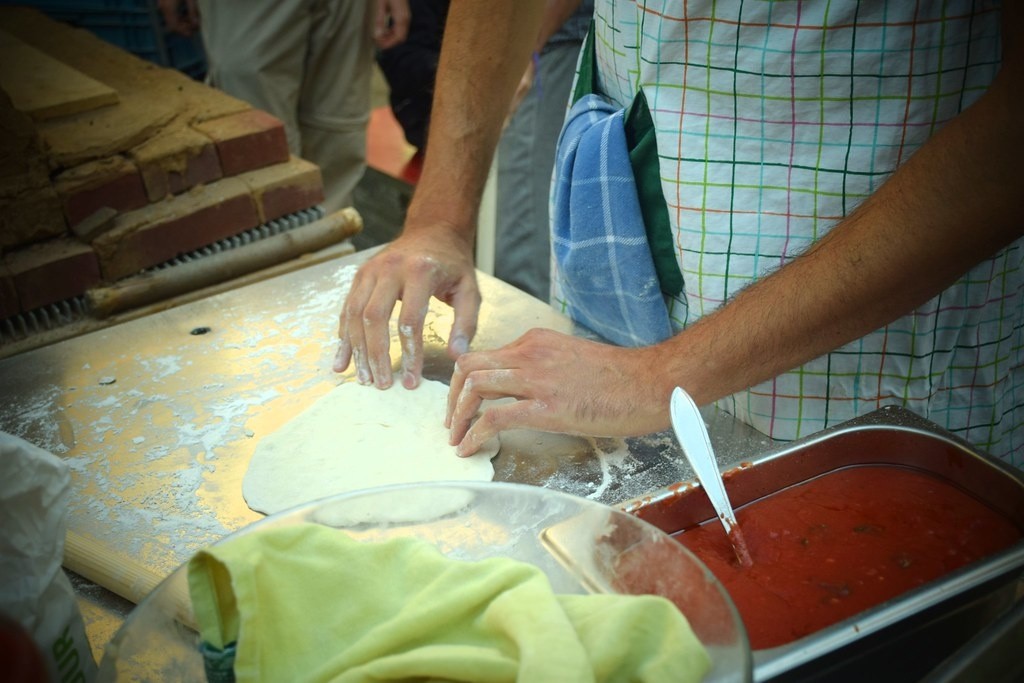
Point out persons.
[332,0,1023,472]
[17,0,593,303]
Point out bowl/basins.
[95,479,754,683]
